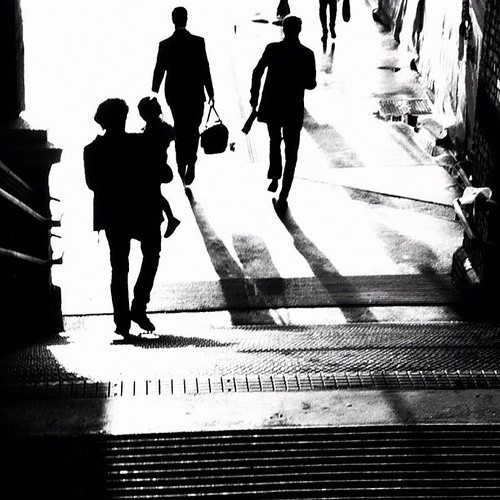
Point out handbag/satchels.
[201,105,228,154]
[342,0,350,22]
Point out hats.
[138,96,162,115]
[94,98,129,124]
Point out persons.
[83,98,173,337]
[152,7,215,185]
[319,0,339,40]
[250,16,317,202]
[138,96,181,238]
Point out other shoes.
[114,326,142,340]
[268,180,279,192]
[164,217,181,238]
[131,306,156,331]
[184,163,195,185]
[330,26,336,38]
